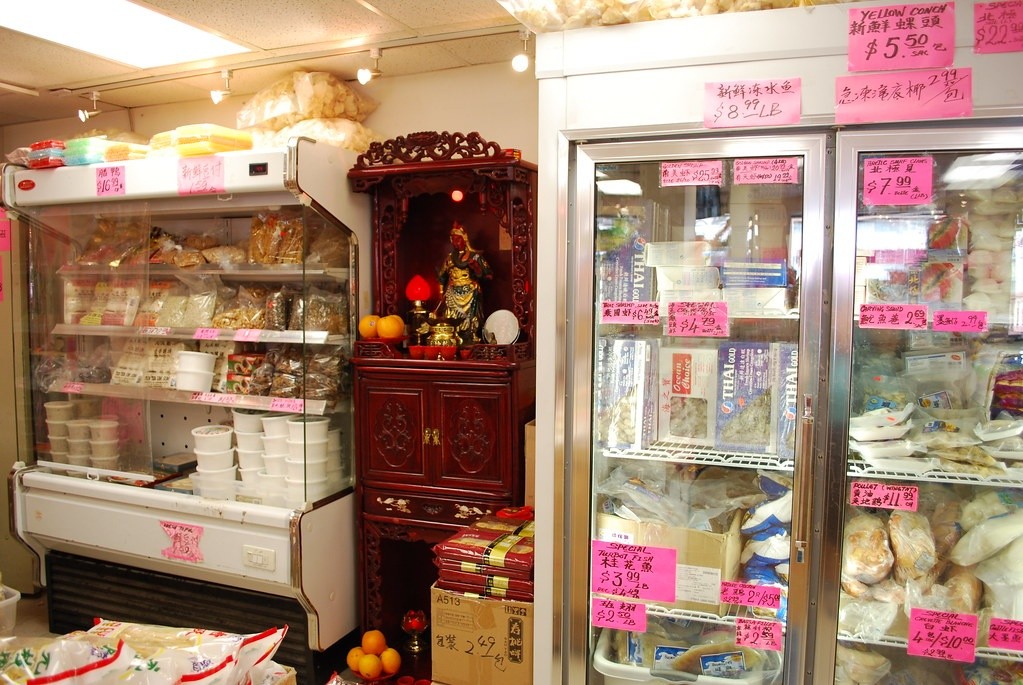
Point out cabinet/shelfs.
[351,359,537,645]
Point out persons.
[438,221,500,344]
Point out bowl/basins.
[176,370,216,394]
[43,396,136,473]
[175,350,218,372]
[190,408,344,500]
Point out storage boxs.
[431,580,534,685]
[857,209,987,440]
[589,242,797,685]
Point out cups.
[408,345,457,360]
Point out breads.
[603,467,1023,685]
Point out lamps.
[401,609,429,653]
[357,48,385,85]
[405,274,432,314]
[512,28,532,72]
[77,90,103,123]
[210,70,234,106]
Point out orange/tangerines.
[347,629,400,679]
[359,314,405,339]
